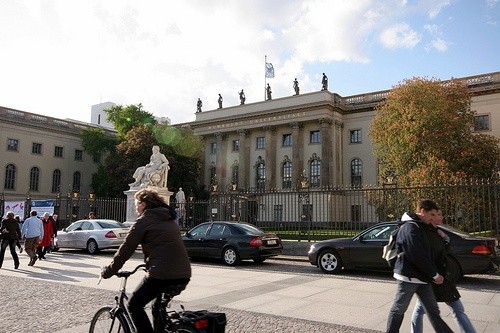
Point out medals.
[293,78,299,95]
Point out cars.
[50,219,130,255]
[307,220,498,283]
[180,221,284,266]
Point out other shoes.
[28,256,37,266]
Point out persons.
[0,210,59,269]
[410,207,478,333]
[85,212,96,220]
[175,188,186,208]
[218,94,222,108]
[321,73,328,91]
[196,98,202,112]
[384,199,456,333]
[100,188,191,333]
[238,89,245,105]
[266,83,272,100]
[128,146,169,187]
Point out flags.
[265,63,274,78]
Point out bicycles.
[88,264,227,333]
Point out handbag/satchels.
[441,253,465,284]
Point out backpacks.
[382,229,398,267]
